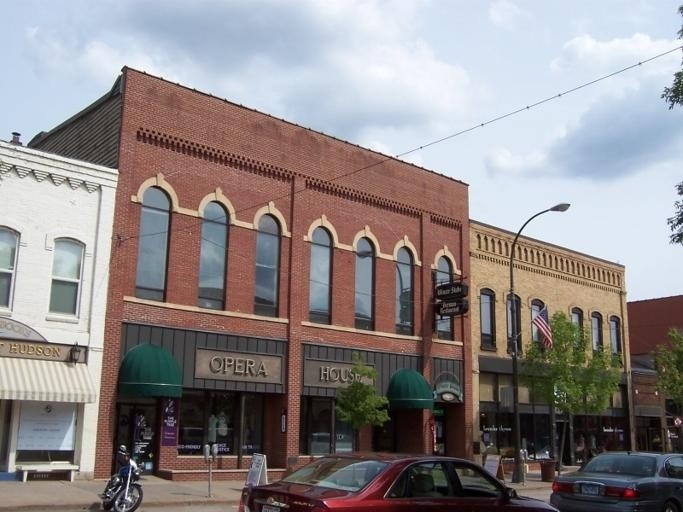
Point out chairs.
[409,473,443,498]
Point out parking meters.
[203,444,219,499]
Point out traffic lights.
[554,389,566,409]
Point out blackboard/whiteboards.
[483,454,501,477]
[245,452,265,486]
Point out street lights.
[508,197,579,477]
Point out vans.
[308,431,353,453]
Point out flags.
[531,306,553,350]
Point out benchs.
[15,464,79,483]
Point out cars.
[549,449,683,512]
[481,428,517,452]
[238,453,560,511]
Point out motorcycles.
[100,444,145,511]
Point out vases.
[536,457,557,483]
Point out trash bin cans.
[540,460,557,482]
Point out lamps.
[69,341,81,365]
